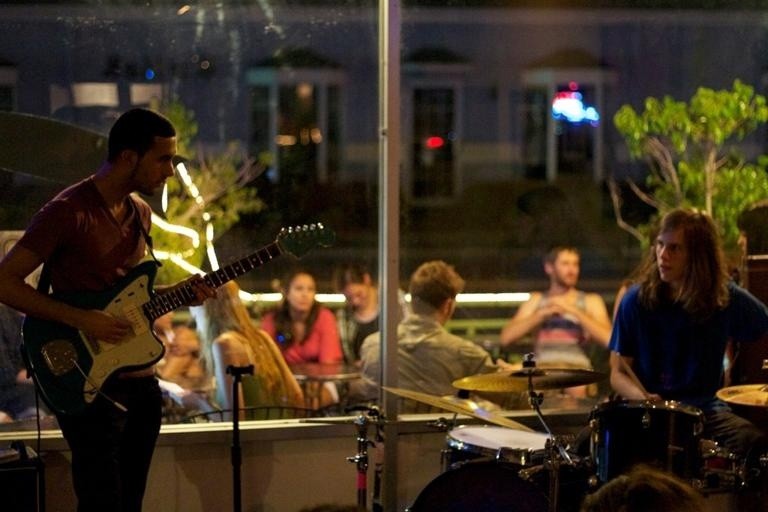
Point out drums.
[406,458,552,510]
[699,447,766,479]
[441,425,558,474]
[531,452,596,487]
[590,397,706,485]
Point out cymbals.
[453,366,601,394]
[381,385,535,434]
[716,384,768,406]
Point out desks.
[169,371,217,394]
[285,359,362,413]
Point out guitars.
[20,221,336,418]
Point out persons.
[581,466,709,511]
[361,260,497,407]
[154,312,202,382]
[604,209,768,467]
[336,265,407,402]
[180,280,301,420]
[498,243,611,398]
[260,272,344,405]
[1,281,54,420]
[1,111,219,512]
